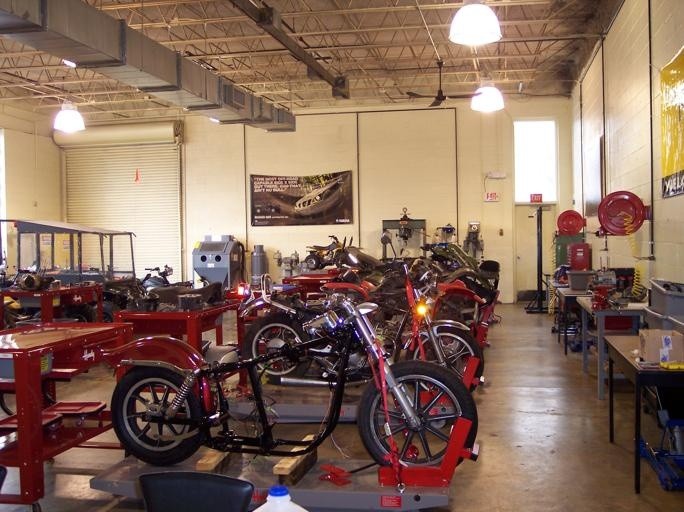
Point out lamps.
[447,1,504,48]
[470,81,506,113]
[53,100,86,133]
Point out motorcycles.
[0,251,194,331]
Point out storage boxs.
[566,270,594,290]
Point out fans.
[407,59,482,107]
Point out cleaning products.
[254,484,308,512]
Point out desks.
[4,287,103,330]
[113,301,248,393]
[546,280,684,494]
[0,319,137,512]
